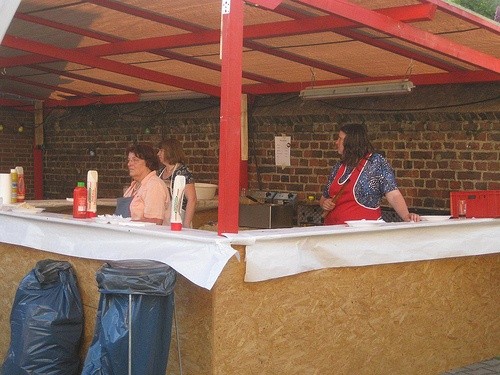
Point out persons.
[319,124,421,226]
[156,138,197,229]
[113,144,170,225]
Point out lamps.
[299,78,416,100]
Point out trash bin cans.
[1,259,86,375]
[78,259,176,374]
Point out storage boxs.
[450,190,500,218]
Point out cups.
[458,200,468,218]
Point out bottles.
[14,166,25,203]
[170,174,186,231]
[86,170,98,218]
[9,169,18,204]
[73,182,88,218]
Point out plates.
[419,214,451,221]
[11,207,45,213]
[344,219,384,227]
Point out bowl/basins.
[195,182,217,199]
